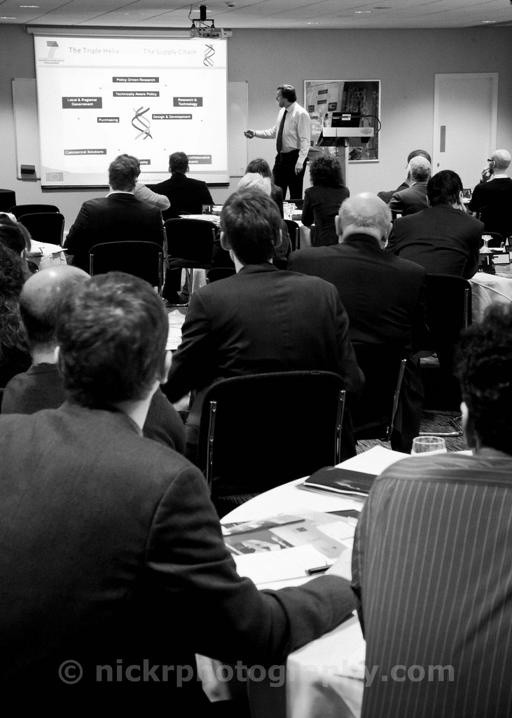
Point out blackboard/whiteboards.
[11,78,250,180]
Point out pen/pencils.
[304,563,333,575]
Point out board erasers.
[19,164,35,182]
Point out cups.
[202,205,210,215]
[283,202,296,220]
[409,436,446,456]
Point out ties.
[275,109,289,154]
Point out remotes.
[244,131,253,138]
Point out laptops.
[331,112,361,127]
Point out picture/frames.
[302,78,380,165]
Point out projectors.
[191,27,232,38]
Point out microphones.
[323,113,328,127]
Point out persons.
[0,147,512,475]
[0,269,358,718]
[244,84,311,200]
[348,302,511,718]
